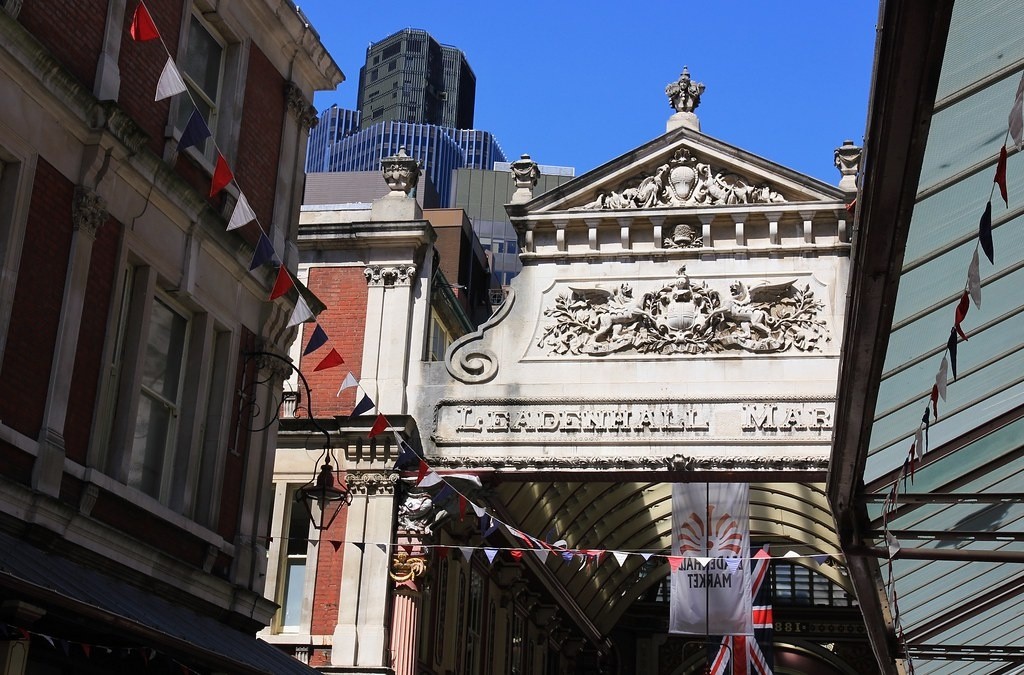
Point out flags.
[669,481,755,635]
[704,544,773,675]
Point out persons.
[665,75,705,115]
